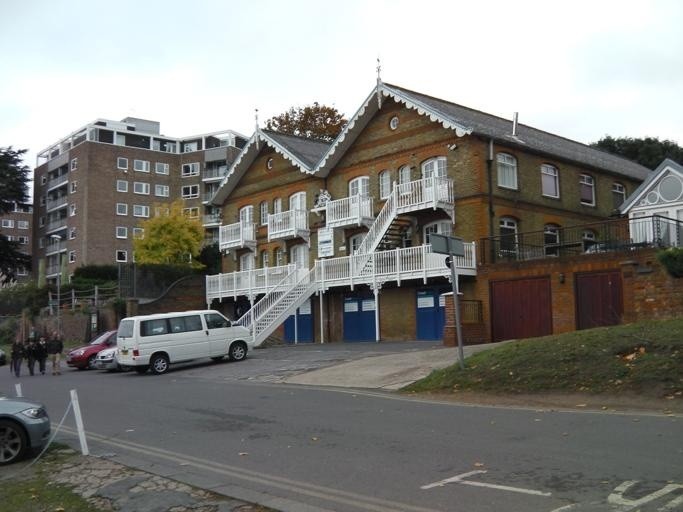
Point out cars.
[0,394,50,463]
[66,329,132,372]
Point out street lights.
[51,235,60,332]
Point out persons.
[10,330,64,377]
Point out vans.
[116,310,252,375]
